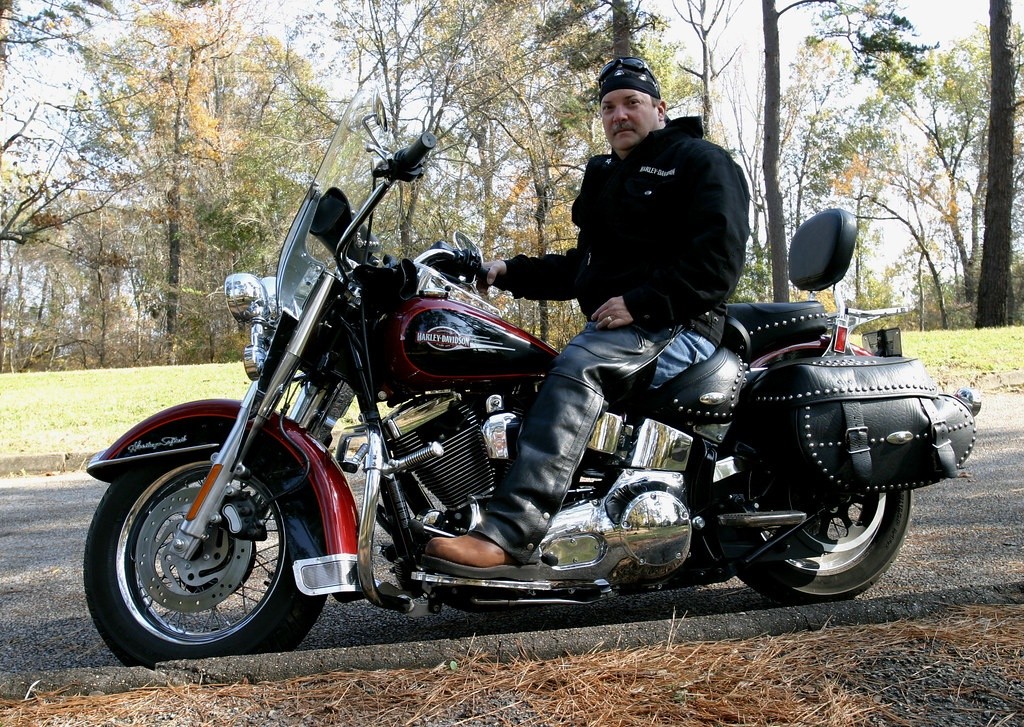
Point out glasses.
[597,57,657,89]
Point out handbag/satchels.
[751,355,977,494]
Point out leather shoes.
[420,530,541,582]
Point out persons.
[420,58,749,579]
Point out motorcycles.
[83,79,984,664]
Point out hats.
[597,57,663,105]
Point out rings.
[607,316,613,322]
